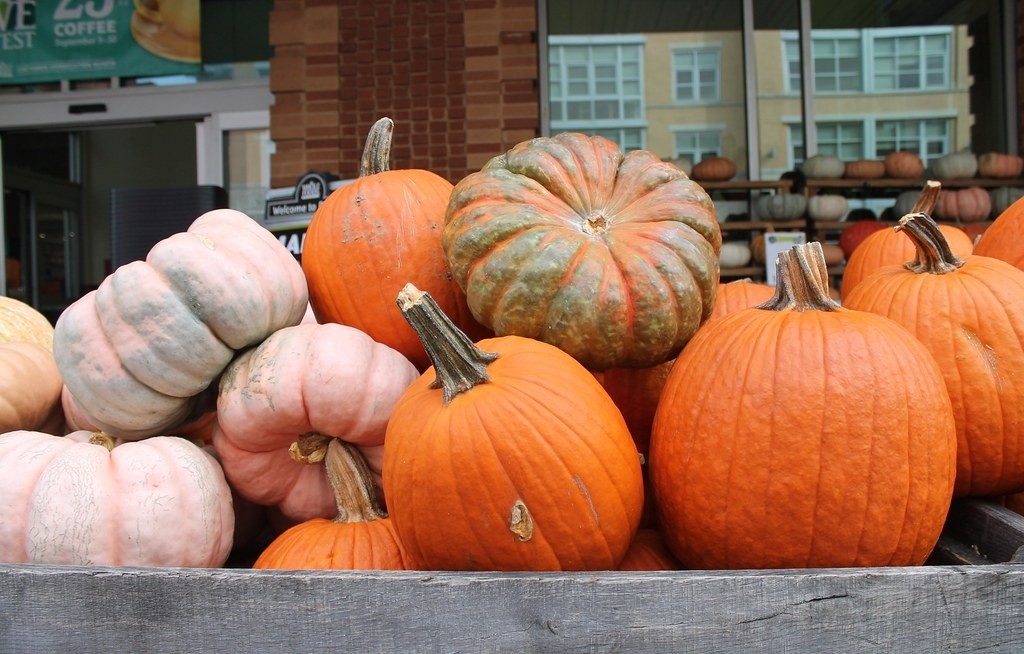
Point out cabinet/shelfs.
[700,177,1024,284]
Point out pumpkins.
[0,115,1024,573]
